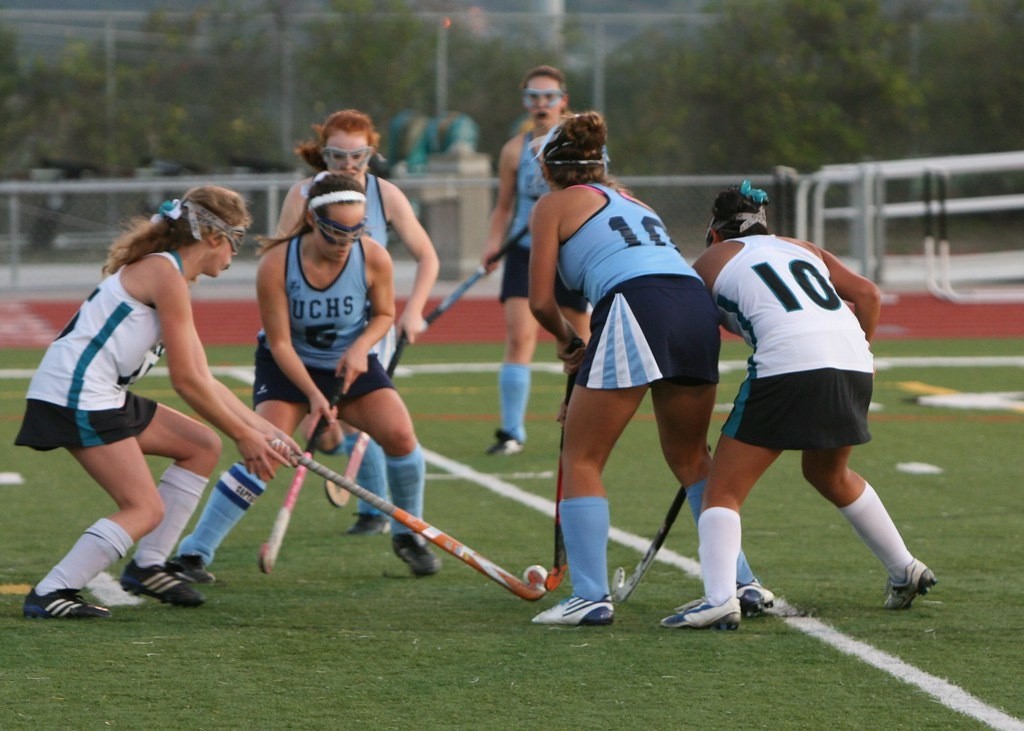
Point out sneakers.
[168,553,216,586]
[674,578,775,618]
[660,593,741,634]
[883,556,938,610]
[23,587,112,621]
[120,559,207,608]
[392,533,442,576]
[346,511,391,537]
[531,592,614,628]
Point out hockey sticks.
[544,338,583,592]
[325,329,409,509]
[423,222,530,331]
[260,367,348,575]
[270,436,549,602]
[608,439,711,603]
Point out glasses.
[227,227,248,252]
[322,145,373,169]
[311,208,368,244]
[521,86,564,108]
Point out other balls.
[523,564,548,585]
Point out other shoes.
[486,429,523,456]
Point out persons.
[529,111,774,627]
[171,174,442,585]
[13,186,303,619]
[662,181,938,630]
[483,65,609,455]
[275,110,439,537]
